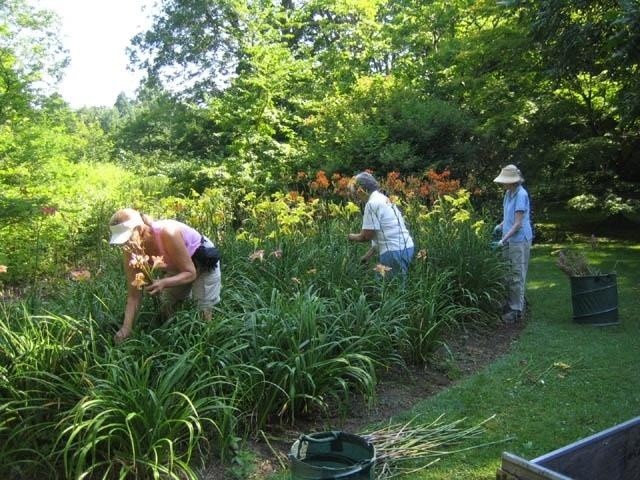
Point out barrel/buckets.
[571,272,619,326]
[289,431,376,480]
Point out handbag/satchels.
[191,234,221,273]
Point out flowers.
[122,233,168,309]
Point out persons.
[491,165,533,324]
[109,208,223,344]
[348,172,414,285]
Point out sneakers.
[500,309,525,325]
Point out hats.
[108,211,144,246]
[492,164,525,185]
